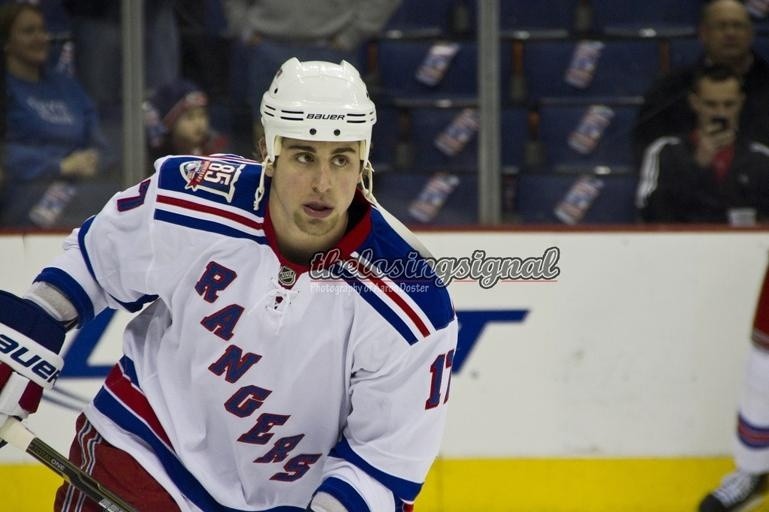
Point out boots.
[698,471,769,512]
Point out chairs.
[361,24,703,220]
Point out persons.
[0,1,405,231]
[631,0,768,225]
[700,251,768,512]
[0,59,458,510]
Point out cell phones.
[712,117,727,137]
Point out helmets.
[260,57,377,167]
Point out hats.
[151,80,208,126]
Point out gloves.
[0,289,66,448]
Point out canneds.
[502,165,519,212]
